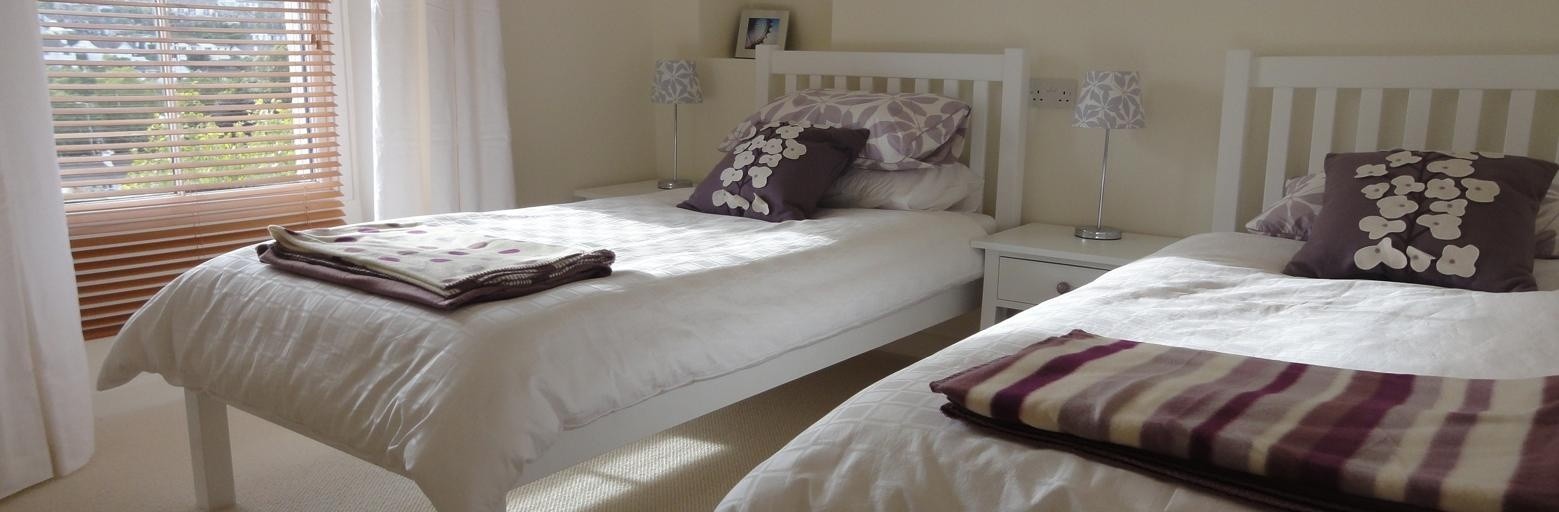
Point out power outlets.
[1028,77,1078,111]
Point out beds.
[717,50,1559,512]
[96,42,1030,512]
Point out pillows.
[821,162,983,213]
[1280,147,1559,293]
[1246,170,1559,260]
[675,121,871,223]
[717,89,971,171]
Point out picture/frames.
[734,9,790,59]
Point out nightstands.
[970,221,1185,333]
[573,178,698,200]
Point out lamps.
[649,57,705,189]
[1069,69,1146,241]
